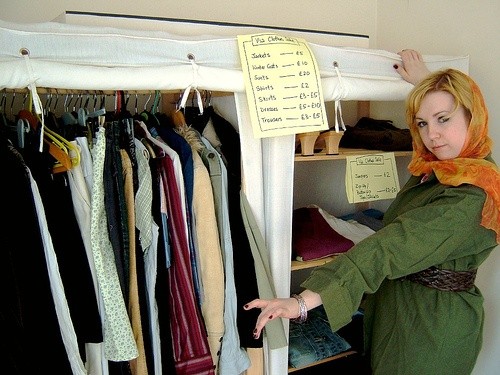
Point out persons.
[244,49,500,375]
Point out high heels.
[295,127,345,155]
[294,132,320,156]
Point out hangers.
[0,88,213,173]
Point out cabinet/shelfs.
[0,10,470,375]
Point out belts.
[399,266,478,292]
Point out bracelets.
[291,293,308,325]
